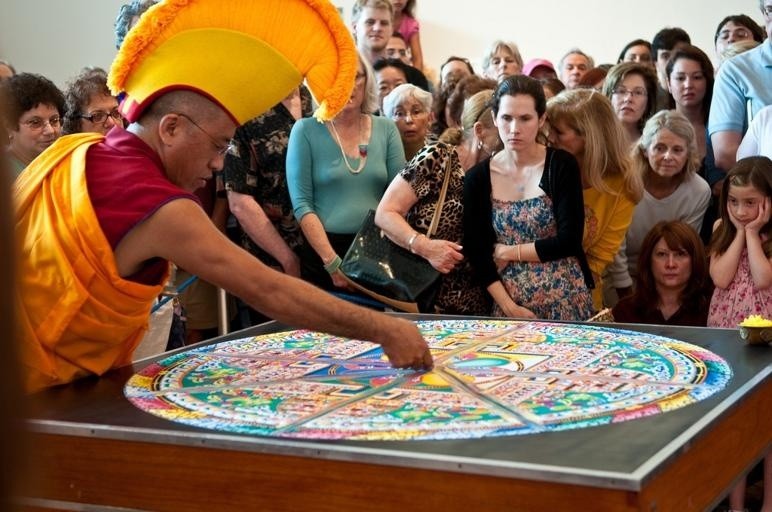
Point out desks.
[10,312,772,510]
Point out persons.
[0,0,770,396]
[704,157,771,511]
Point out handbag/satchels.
[338,209,451,313]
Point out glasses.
[20,118,61,131]
[83,110,122,124]
[180,114,231,154]
[716,31,749,41]
[612,87,647,97]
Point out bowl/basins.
[736,323,772,346]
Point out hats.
[107,0,357,127]
[522,59,555,77]
